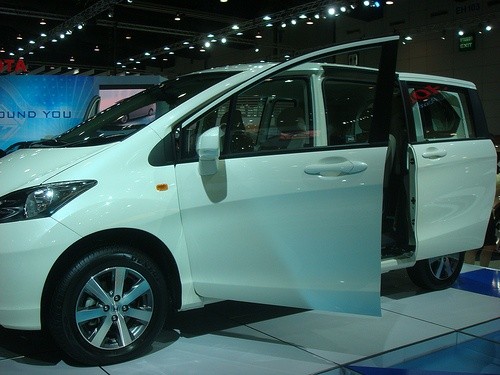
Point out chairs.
[215,110,252,152]
[257,107,304,152]
[353,110,396,189]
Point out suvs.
[0,34,498,366]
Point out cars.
[120,102,156,124]
